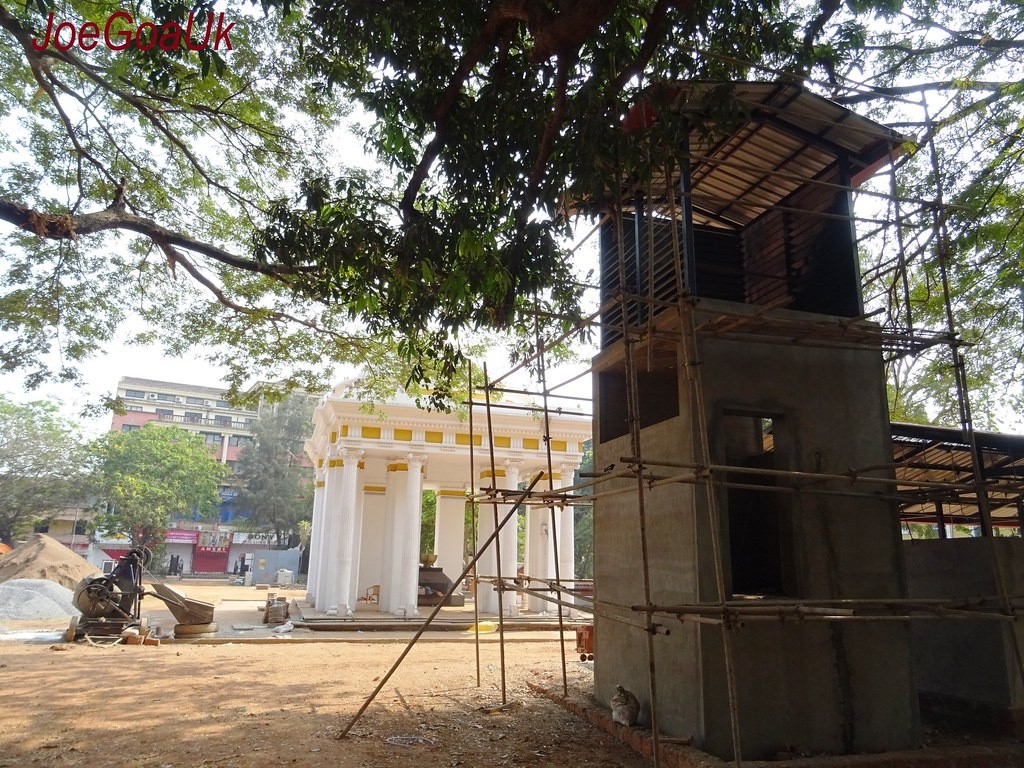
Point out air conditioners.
[175,396,182,402]
[149,393,158,399]
[204,400,211,405]
